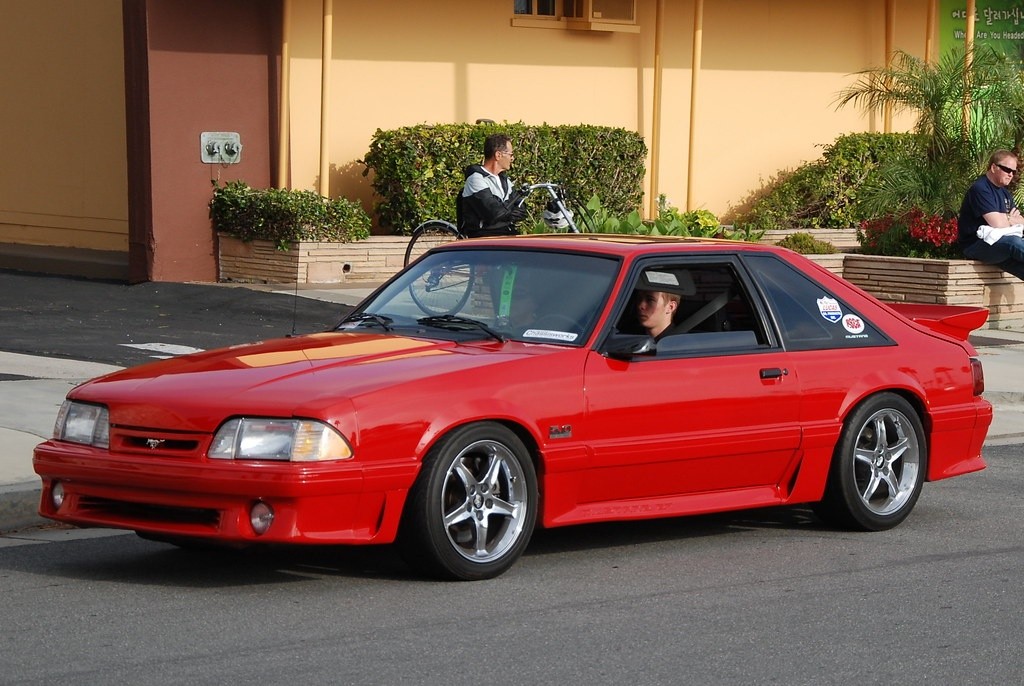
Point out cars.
[32,233,993,583]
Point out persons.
[455,133,534,271]
[609,272,693,360]
[490,263,539,332]
[957,149,1024,281]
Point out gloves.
[511,208,528,222]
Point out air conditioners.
[560,0,639,25]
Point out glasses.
[996,164,1016,175]
[501,151,514,157]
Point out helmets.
[543,208,574,228]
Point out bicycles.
[403,179,597,319]
